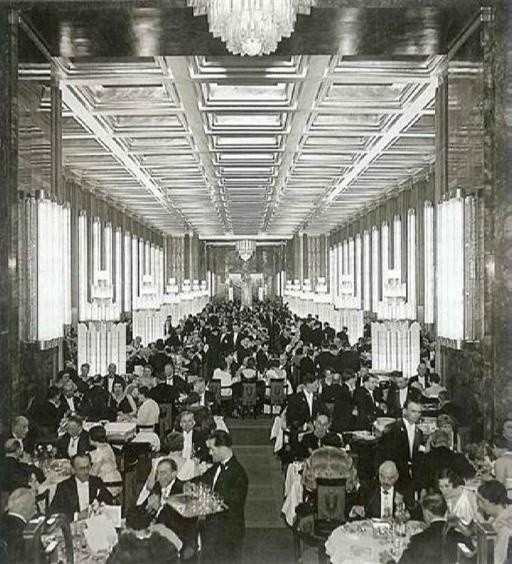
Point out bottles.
[371,501,409,553]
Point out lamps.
[29,186,211,376]
[236,241,257,259]
[278,181,471,380]
[183,0,319,54]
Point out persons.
[2,295,512,563]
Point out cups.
[198,481,225,514]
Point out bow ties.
[383,490,389,495]
[218,462,227,471]
